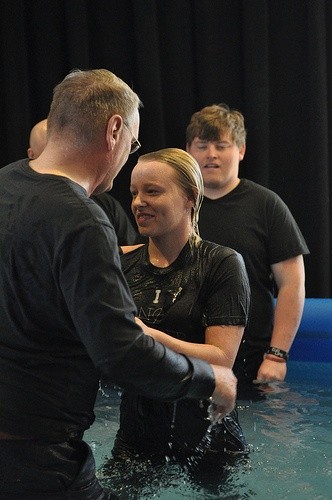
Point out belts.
[0,432,78,444]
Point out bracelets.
[263,346,289,365]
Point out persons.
[0,69,251,500]
[28,105,309,500]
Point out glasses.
[123,122,141,154]
